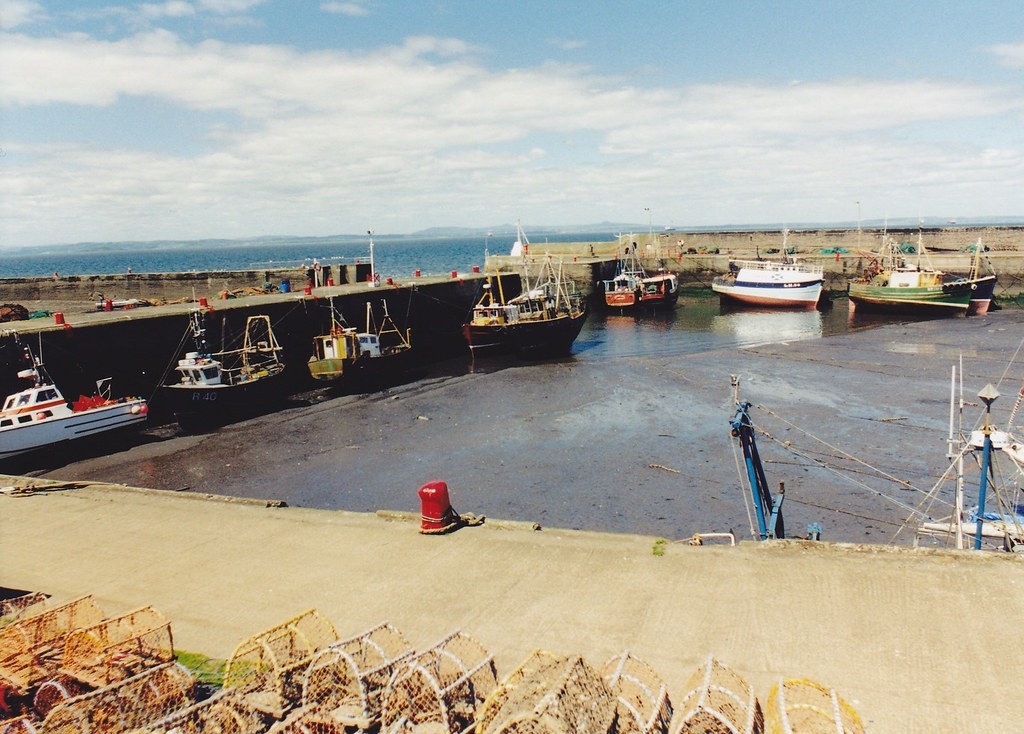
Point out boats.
[604,230,680,312]
[308,281,417,390]
[711,227,824,311]
[159,287,286,427]
[466,217,589,368]
[665,338,1024,553]
[0,331,148,459]
[847,227,997,318]
